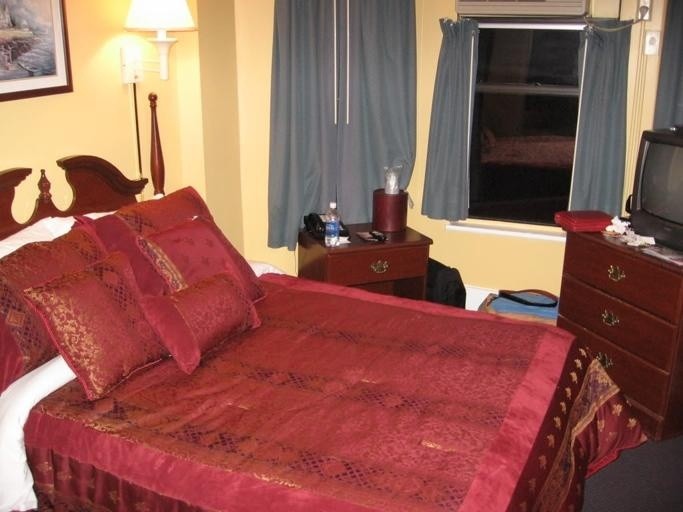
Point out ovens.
[1,153,591,508]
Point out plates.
[556,221,682,440]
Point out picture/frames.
[1,0,73,102]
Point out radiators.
[369,230,387,242]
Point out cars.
[382,162,404,194]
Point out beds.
[1,153,591,508]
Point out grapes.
[296,225,434,300]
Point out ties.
[630,128,683,252]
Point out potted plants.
[1,0,73,102]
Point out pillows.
[87,188,215,250]
[21,251,168,401]
[121,218,265,302]
[137,271,260,376]
[4,223,112,384]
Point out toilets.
[304,212,348,238]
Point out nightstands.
[296,225,434,300]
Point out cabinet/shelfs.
[325,201,339,247]
[556,221,682,440]
